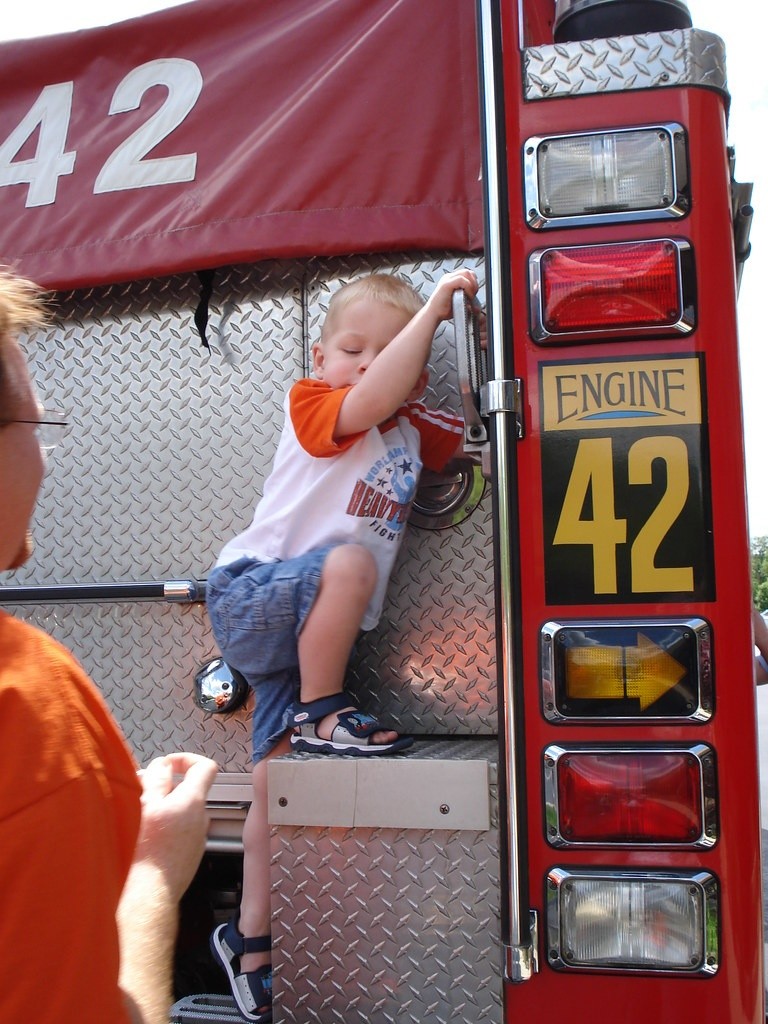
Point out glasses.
[1,409,67,449]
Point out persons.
[754,609,768,686]
[207,269,483,1023]
[0,270,220,1024]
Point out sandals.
[211,907,275,1024]
[283,691,416,758]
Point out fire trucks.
[0,0,768,1023]
